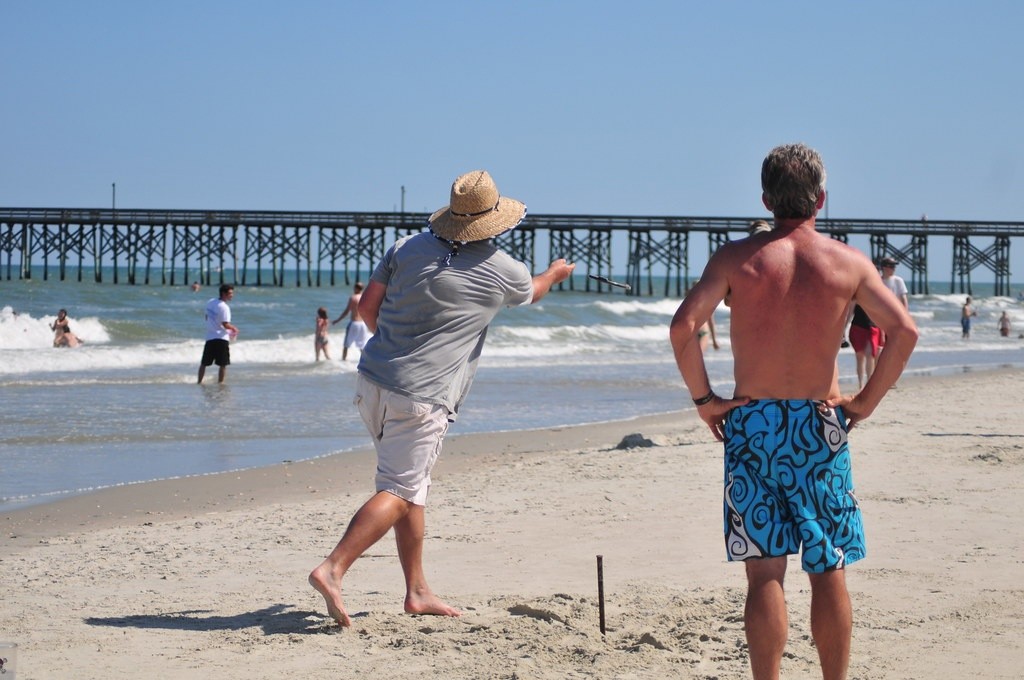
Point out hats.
[426,170,528,267]
[880,257,900,265]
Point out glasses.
[886,265,896,269]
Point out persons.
[307,170,575,629]
[193,281,199,291]
[52,309,80,347]
[332,282,367,360]
[1018,292,1024,301]
[841,257,909,391]
[198,286,237,384]
[961,296,978,338]
[315,308,330,360]
[669,143,918,680]
[685,279,719,352]
[996,311,1011,336]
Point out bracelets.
[693,390,714,406]
